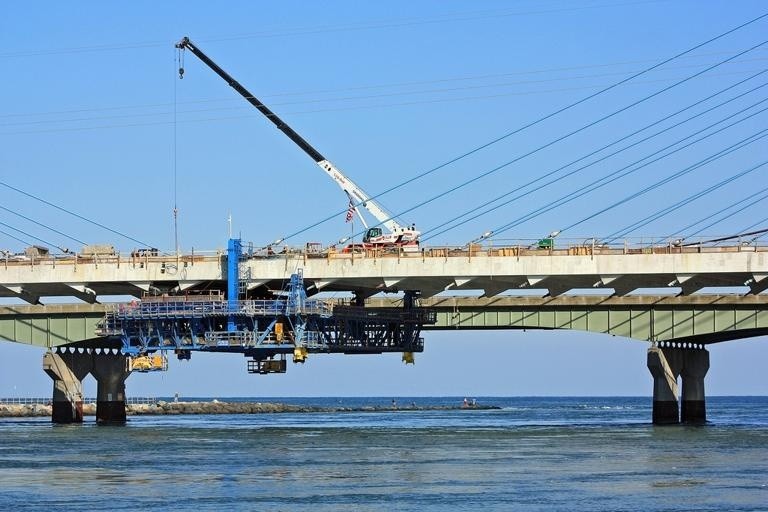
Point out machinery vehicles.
[176,35,421,252]
